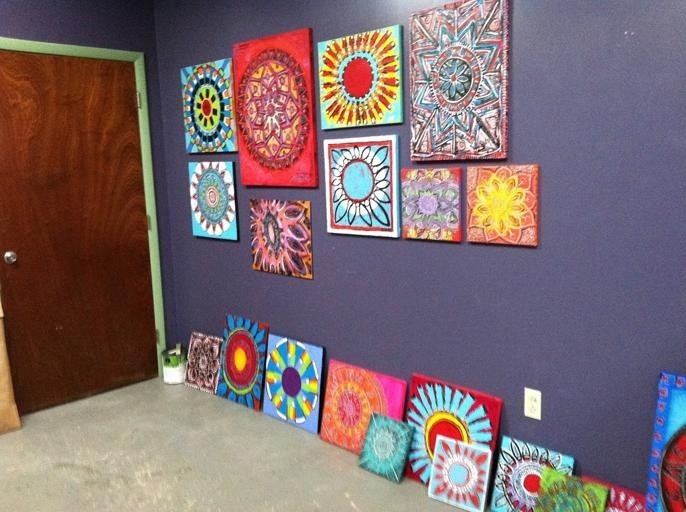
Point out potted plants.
[161,347,189,387]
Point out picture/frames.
[427,432,494,507]
[249,196,314,281]
[232,27,319,191]
[405,370,502,501]
[647,373,686,512]
[467,164,542,249]
[185,160,239,245]
[399,166,463,243]
[491,432,575,512]
[536,465,609,512]
[323,135,400,240]
[579,472,647,512]
[184,330,221,393]
[406,0,508,162]
[319,356,408,465]
[217,310,267,418]
[263,332,326,440]
[315,23,407,133]
[357,413,416,485]
[180,56,239,158]
